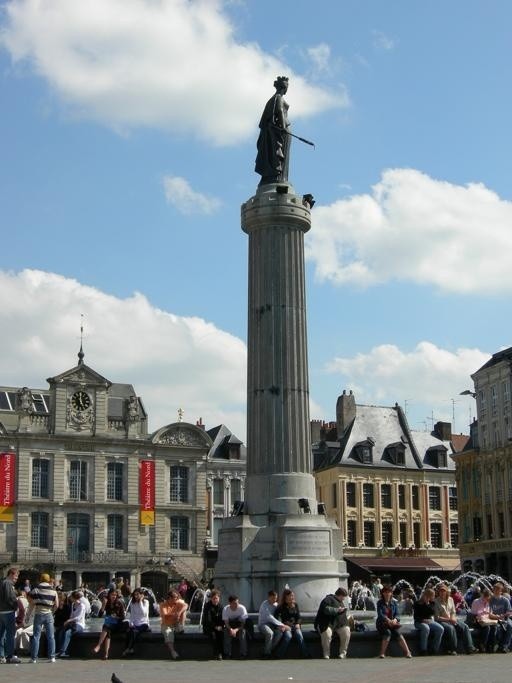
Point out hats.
[438,584,448,592]
[40,573,50,583]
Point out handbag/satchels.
[381,620,402,630]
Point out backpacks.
[228,615,245,630]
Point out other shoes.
[50,658,56,663]
[92,646,134,659]
[0,656,6,663]
[380,654,384,658]
[6,655,21,663]
[212,652,249,660]
[167,650,179,660]
[324,656,329,659]
[31,659,36,663]
[259,653,313,660]
[339,654,345,659]
[419,645,510,655]
[406,655,412,658]
[55,651,70,657]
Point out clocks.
[70,389,92,412]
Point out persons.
[255,77,293,185]
